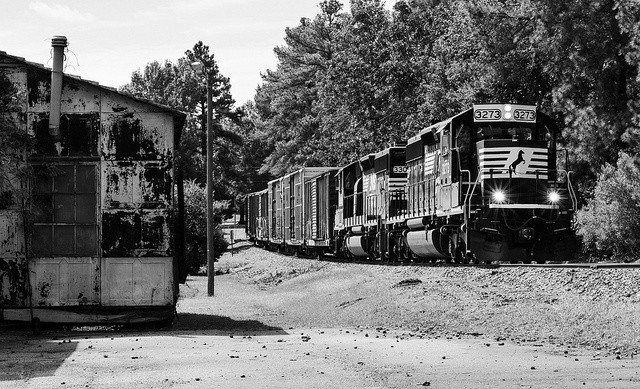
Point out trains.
[243,103,583,264]
